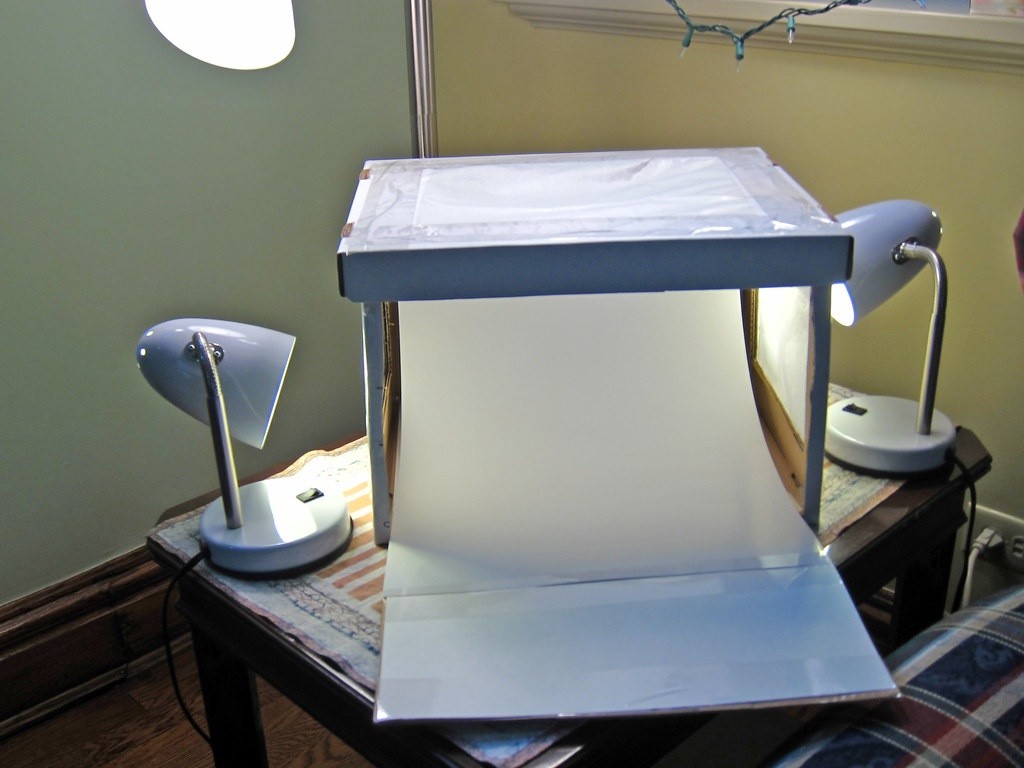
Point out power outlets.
[962,502,1024,568]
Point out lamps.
[824,199,957,476]
[133,319,350,573]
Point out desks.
[144,385,968,768]
